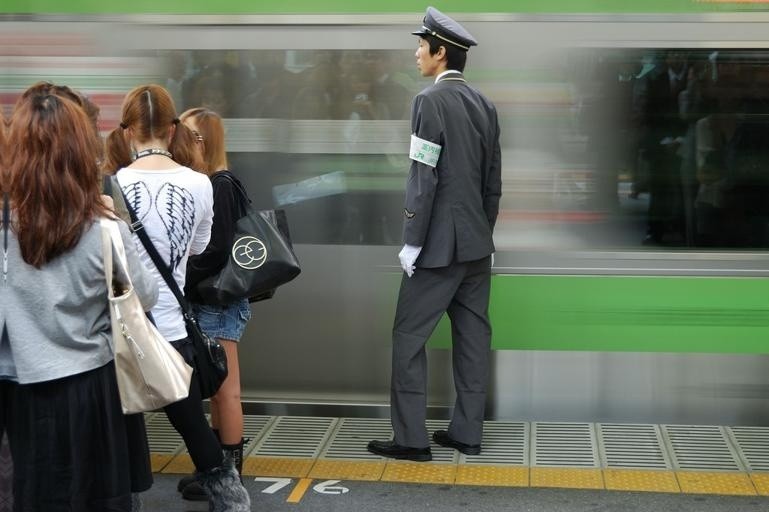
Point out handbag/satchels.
[194,172,302,308]
[94,192,193,415]
[147,336,229,414]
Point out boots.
[176,427,250,501]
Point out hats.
[411,6,478,51]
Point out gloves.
[398,244,423,278]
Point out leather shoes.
[431,430,481,456]
[367,439,433,462]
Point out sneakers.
[639,231,697,249]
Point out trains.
[0,0,768,425]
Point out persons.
[366,5,501,461]
[1,81,253,512]
